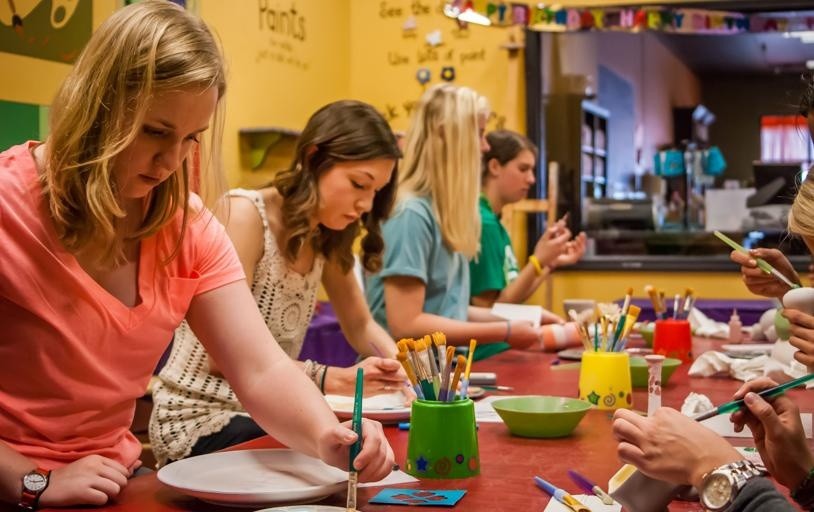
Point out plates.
[156,443,364,503]
[311,390,445,422]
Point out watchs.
[696,458,771,510]
[15,464,55,506]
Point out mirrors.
[525,0,814,272]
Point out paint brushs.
[647,286,693,320]
[396,331,477,400]
[480,385,512,390]
[568,287,641,352]
[534,476,591,512]
[695,373,814,422]
[569,470,614,505]
[713,230,800,289]
[399,422,410,429]
[369,341,411,388]
[347,367,364,512]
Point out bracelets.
[310,361,324,383]
[301,359,312,376]
[503,317,513,344]
[527,251,541,278]
[320,363,330,396]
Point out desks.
[24,336,814,512]
[616,298,774,327]
[301,302,359,367]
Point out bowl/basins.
[490,391,594,439]
[629,356,683,385]
[640,323,699,349]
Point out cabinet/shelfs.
[546,97,610,233]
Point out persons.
[609,379,813,510]
[0,1,398,505]
[794,71,813,150]
[145,100,416,470]
[360,82,564,367]
[469,125,588,307]
[730,162,814,373]
